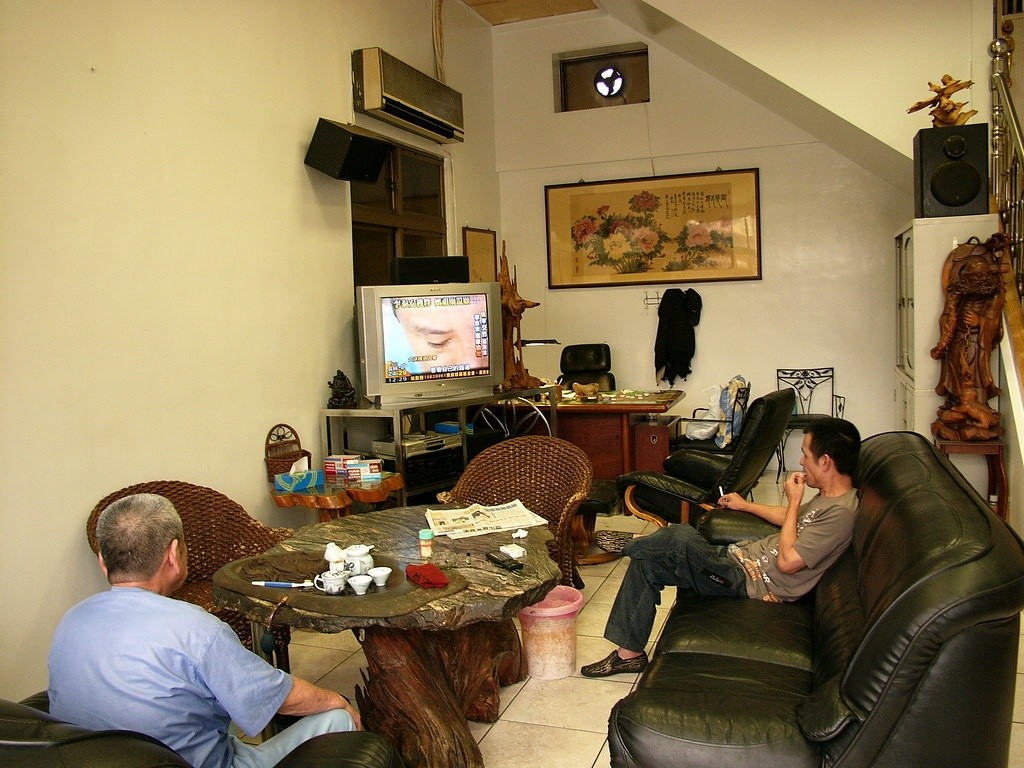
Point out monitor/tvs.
[356,282,505,408]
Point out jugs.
[344,543,375,578]
[313,570,351,595]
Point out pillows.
[686,384,722,441]
[714,375,747,450]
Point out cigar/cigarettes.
[719,486,725,502]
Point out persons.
[390,295,489,373]
[930,257,1004,413]
[48,493,362,768]
[582,418,862,677]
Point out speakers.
[390,255,470,284]
[304,119,391,183]
[913,123,988,218]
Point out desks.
[932,435,1008,520]
[270,470,405,521]
[464,390,686,479]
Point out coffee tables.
[213,505,557,767]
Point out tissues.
[275,455,324,491]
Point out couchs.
[607,431,1024,768]
[0,689,399,768]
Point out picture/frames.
[462,226,497,282]
[544,166,762,289]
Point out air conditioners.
[350,47,465,146]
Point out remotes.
[486,550,524,572]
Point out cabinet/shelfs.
[892,213,1005,504]
[324,395,499,507]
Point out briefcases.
[265,424,311,482]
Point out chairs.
[775,367,846,484]
[85,479,297,663]
[556,343,616,390]
[614,387,796,528]
[670,384,754,502]
[451,436,594,590]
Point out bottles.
[419,528,434,558]
[990,495,998,513]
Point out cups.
[368,567,392,587]
[348,575,372,595]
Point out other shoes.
[594,530,649,556]
[581,649,650,677]
